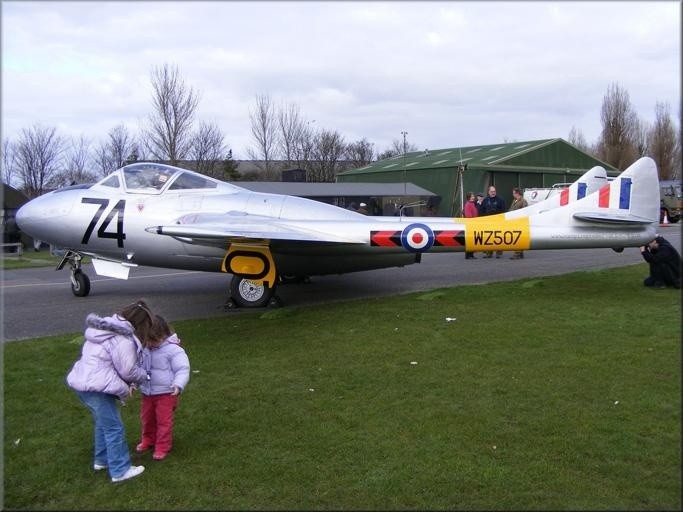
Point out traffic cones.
[662,211,669,226]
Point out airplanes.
[13,161,666,309]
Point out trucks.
[658,184,683,223]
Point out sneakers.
[112,466,145,482]
[94,464,108,470]
[465,255,524,260]
[153,451,169,459]
[137,443,152,451]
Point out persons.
[356,202,367,215]
[640,236,683,289]
[508,187,528,259]
[476,193,486,257]
[478,185,505,258]
[346,202,356,211]
[463,192,479,259]
[4,214,20,253]
[33,239,42,252]
[65,300,151,483]
[129,314,191,460]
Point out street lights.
[399,131,410,205]
